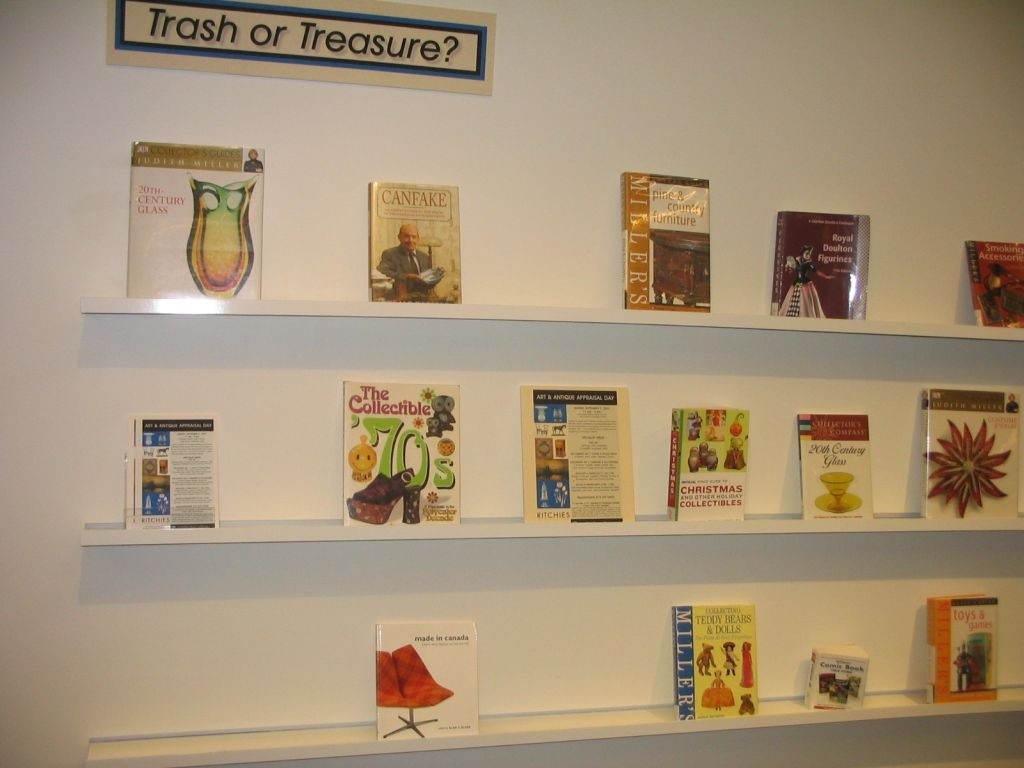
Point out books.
[127,416,219,532]
[807,648,870,711]
[620,172,713,314]
[929,594,999,702]
[798,413,875,521]
[673,603,759,723]
[668,409,751,521]
[919,386,1024,525]
[967,241,1024,330]
[343,379,463,527]
[377,622,477,739]
[369,181,462,307]
[772,211,871,321]
[520,385,636,526]
[127,142,266,300]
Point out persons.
[243,149,263,172]
[376,224,440,303]
[778,246,835,319]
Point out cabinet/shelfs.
[76,308,1024,768]
